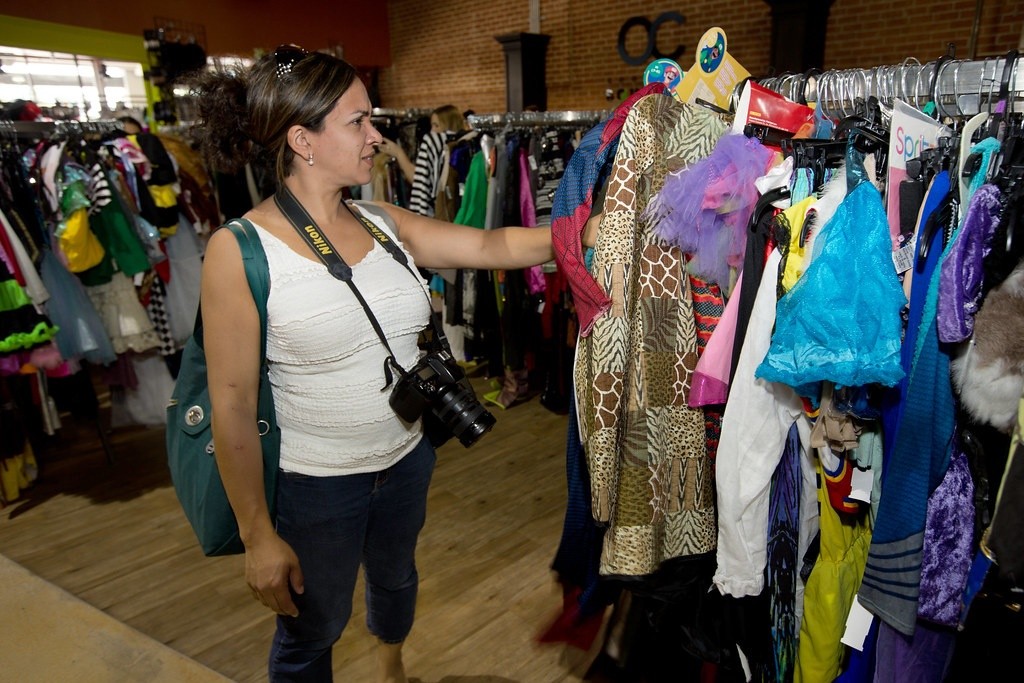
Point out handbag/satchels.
[165,215,283,558]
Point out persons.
[201,44,603,683]
[377,106,464,185]
[118,117,145,134]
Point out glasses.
[274,44,309,93]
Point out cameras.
[388,345,497,448]
